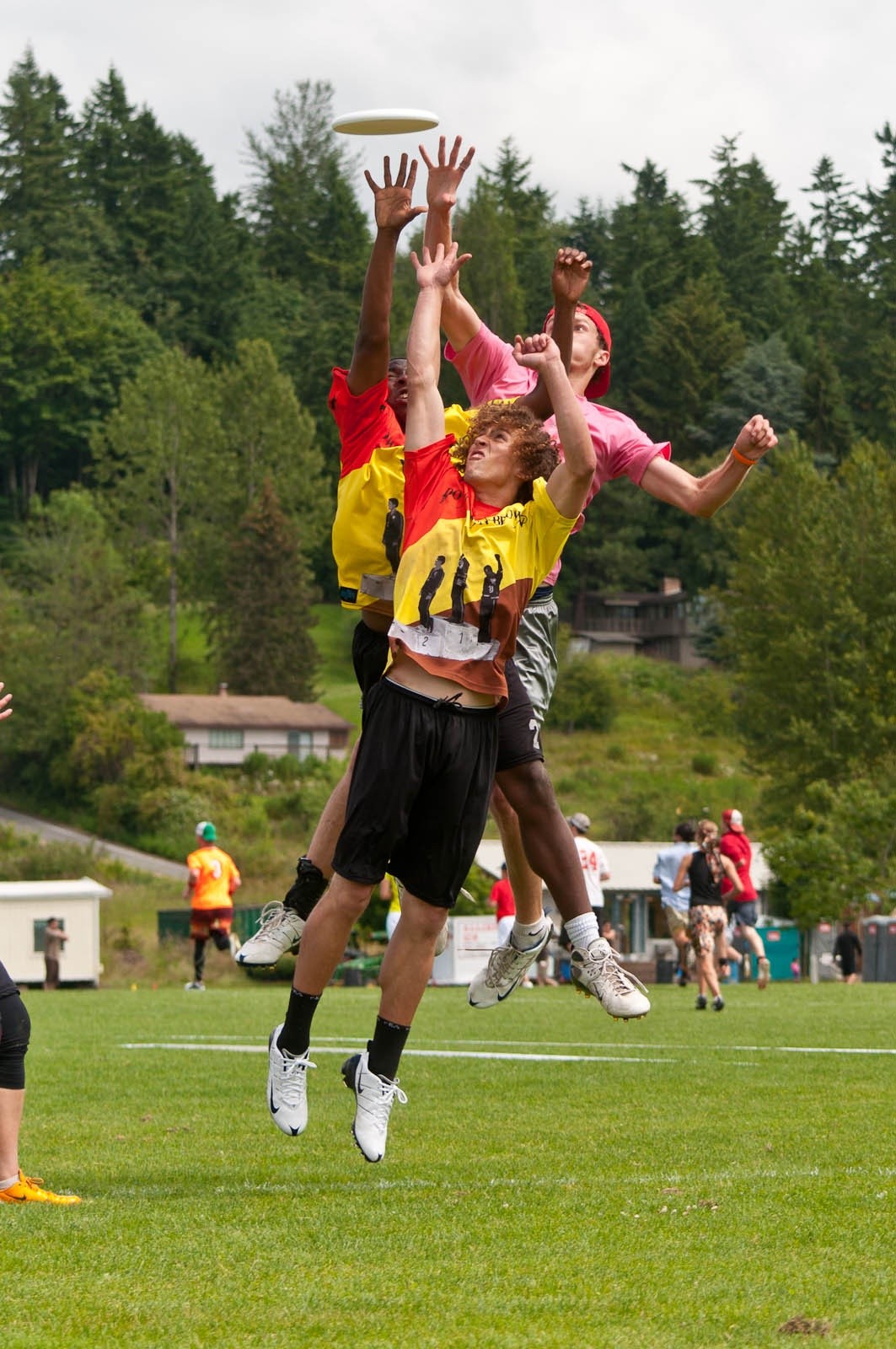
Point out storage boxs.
[431,915,502,986]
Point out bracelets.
[730,447,757,466]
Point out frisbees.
[460,887,476,903]
[332,109,437,135]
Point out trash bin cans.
[558,958,572,983]
[656,960,674,983]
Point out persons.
[0,961,82,1206]
[832,922,861,983]
[791,957,801,982]
[418,555,446,633]
[717,808,771,989]
[43,918,68,991]
[488,861,560,988]
[477,553,503,643]
[182,820,242,990]
[443,553,470,624]
[672,819,744,1013]
[268,239,595,1164]
[382,498,404,581]
[652,822,701,983]
[601,922,617,947]
[236,153,594,980]
[419,129,778,1023]
[381,867,405,940]
[557,812,612,950]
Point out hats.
[543,302,617,400]
[496,862,508,871]
[564,812,591,832]
[720,807,748,833]
[194,821,218,842]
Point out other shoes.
[184,980,207,991]
[229,932,242,957]
[677,969,692,986]
[713,996,725,1010]
[682,940,695,956]
[738,953,752,980]
[695,996,708,1010]
[716,965,732,982]
[754,959,772,990]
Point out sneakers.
[266,1023,320,1136]
[1,1170,83,1206]
[233,899,306,970]
[394,877,451,956]
[340,1048,408,1163]
[465,915,557,1008]
[569,937,651,1022]
